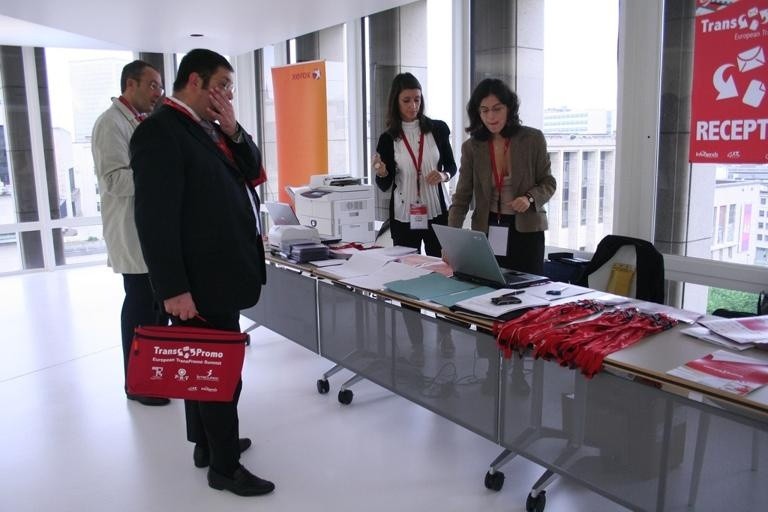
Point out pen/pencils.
[503,291,525,297]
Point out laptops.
[264,201,342,242]
[431,224,549,287]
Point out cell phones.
[491,297,521,305]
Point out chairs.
[583,234,664,301]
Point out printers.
[286,174,375,242]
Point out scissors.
[547,288,568,295]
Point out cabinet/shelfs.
[294,184,375,243]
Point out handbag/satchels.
[126,314,249,403]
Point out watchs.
[523,192,535,204]
[445,171,451,181]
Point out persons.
[373,72,457,367]
[440,79,556,394]
[129,50,274,495]
[92,60,172,406]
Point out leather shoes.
[511,370,530,395]
[413,350,424,366]
[481,370,497,396]
[127,392,171,406]
[193,438,275,496]
[439,335,455,356]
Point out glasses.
[136,80,164,96]
[211,77,237,95]
[479,104,505,113]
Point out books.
[665,315,768,397]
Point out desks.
[239,230,766,512]
